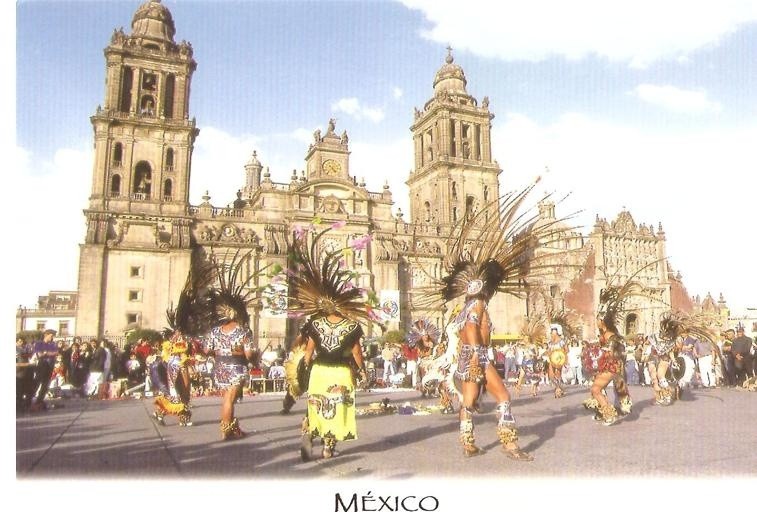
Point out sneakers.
[301,435,312,462]
[591,410,629,427]
[279,409,288,414]
[321,446,340,459]
[151,412,164,426]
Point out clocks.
[322,159,342,177]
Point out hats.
[595,312,611,321]
[466,280,487,302]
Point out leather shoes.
[504,442,534,461]
[464,444,487,458]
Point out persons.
[149,329,194,426]
[202,307,258,442]
[450,281,532,459]
[16,323,756,414]
[295,310,371,463]
[589,312,632,424]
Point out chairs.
[247,365,287,393]
[493,349,505,373]
